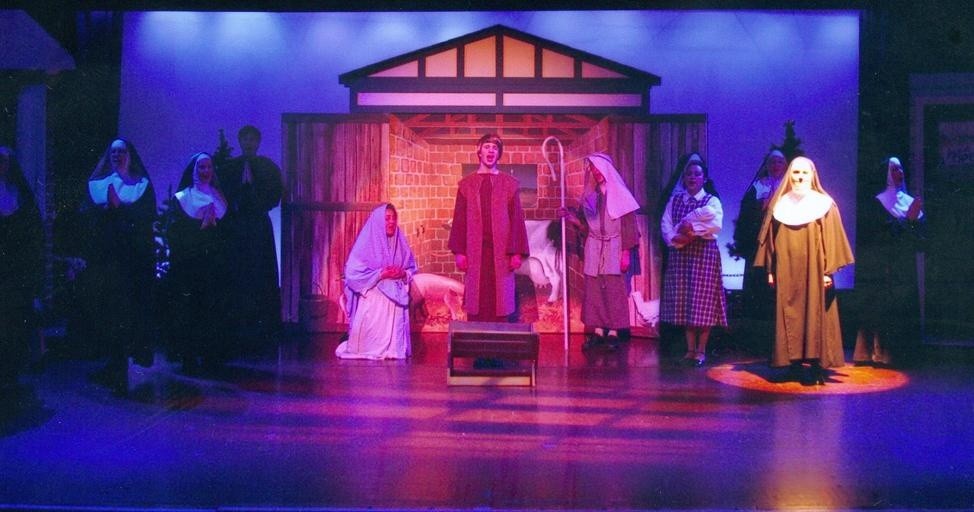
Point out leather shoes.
[580,333,621,354]
[677,349,707,370]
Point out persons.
[168,151,253,373]
[72,136,158,370]
[0,145,46,418]
[660,154,728,369]
[333,203,415,359]
[854,155,930,368]
[730,149,790,357]
[751,155,856,386]
[555,152,642,352]
[448,130,530,370]
[215,124,282,319]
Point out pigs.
[407,271,468,324]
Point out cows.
[442,213,580,305]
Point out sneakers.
[792,362,819,387]
[168,352,242,386]
[853,351,893,370]
[90,364,132,398]
[470,358,503,371]
